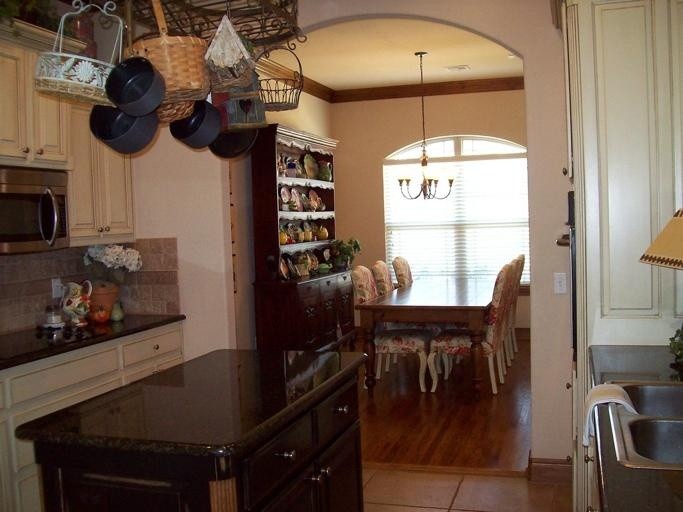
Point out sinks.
[625,417,683,466]
[621,382,683,417]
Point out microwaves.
[0,166,72,255]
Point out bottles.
[45,328,65,349]
[42,304,66,330]
[110,301,125,322]
[113,320,124,335]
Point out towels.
[579,381,638,446]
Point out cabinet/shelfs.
[0,35,69,165]
[13,347,370,511]
[120,324,183,386]
[69,52,135,250]
[249,123,336,276]
[253,266,354,363]
[0,343,120,510]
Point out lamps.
[396,50,454,201]
[638,205,683,377]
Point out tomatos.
[89,306,109,324]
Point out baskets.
[210,49,257,93]
[32,4,122,108]
[123,0,212,123]
[251,47,304,110]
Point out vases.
[84,287,116,320]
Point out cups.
[298,230,312,242]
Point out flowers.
[79,243,144,287]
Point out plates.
[280,253,319,277]
[287,220,319,239]
[280,187,320,211]
[286,153,320,177]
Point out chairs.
[447,259,519,376]
[392,255,411,289]
[348,265,428,393]
[372,260,443,374]
[427,263,517,396]
[511,254,525,357]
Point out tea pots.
[316,225,329,239]
[59,279,92,327]
[64,326,94,348]
[318,161,333,181]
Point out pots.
[89,55,259,159]
